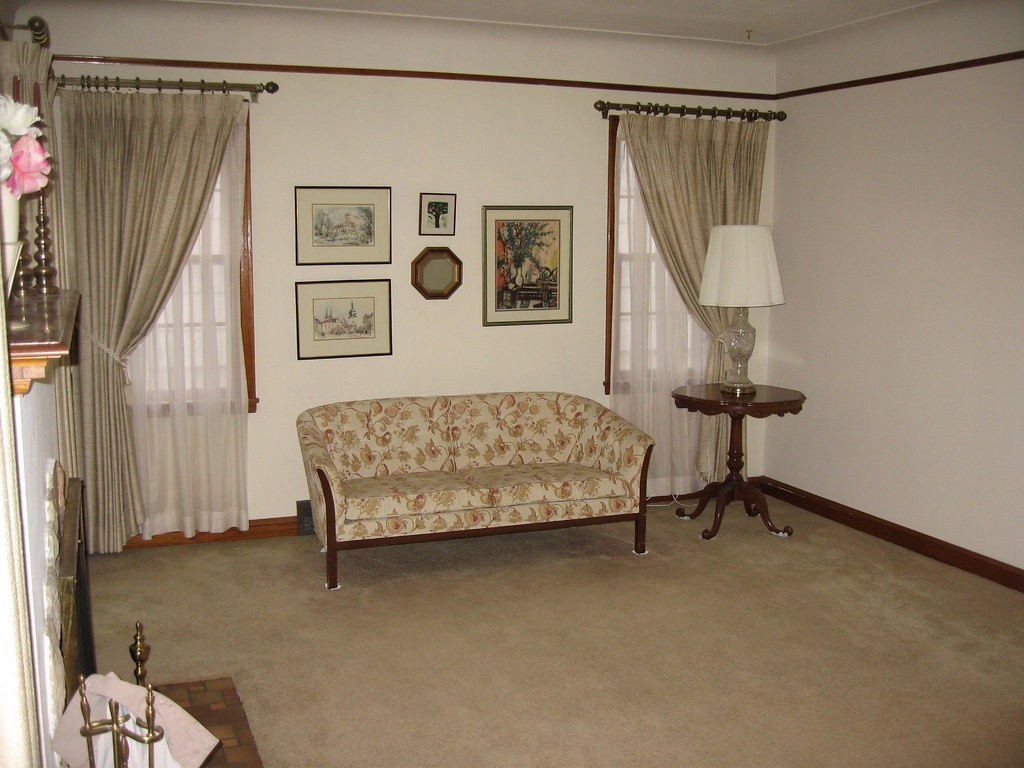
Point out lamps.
[698,225,786,394]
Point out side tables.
[671,384,806,541]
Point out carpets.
[152,676,264,768]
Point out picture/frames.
[419,193,456,235]
[295,278,392,360]
[294,186,391,265]
[482,205,573,327]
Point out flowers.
[0,92,52,201]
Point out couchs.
[296,391,656,590]
